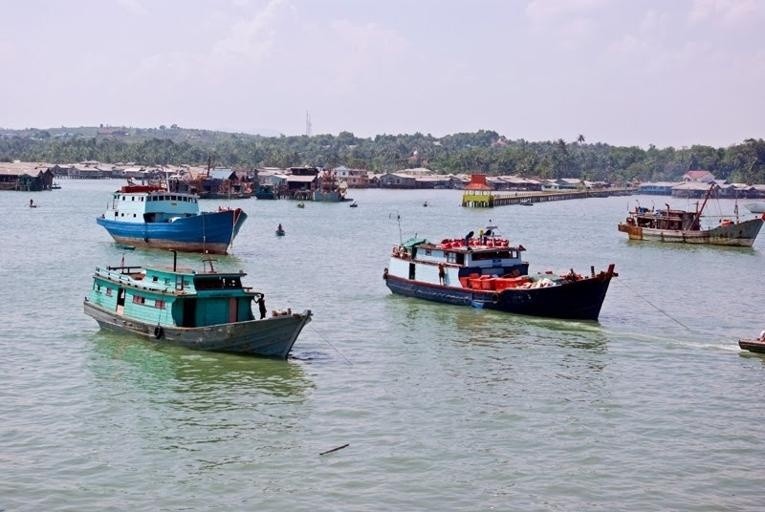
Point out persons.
[438,263,446,286]
[254,294,267,319]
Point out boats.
[94,174,249,257]
[378,206,617,326]
[82,242,313,362]
[616,180,765,251]
